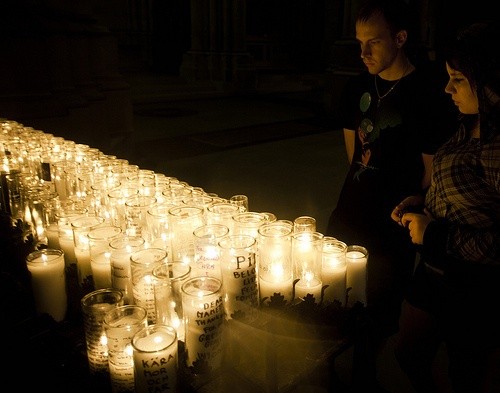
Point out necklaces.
[374,65,411,106]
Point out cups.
[0,116,369,393]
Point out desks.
[190,301,368,393]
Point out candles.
[0,122,368,393]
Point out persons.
[390,22,500,393]
[325,0,454,393]
